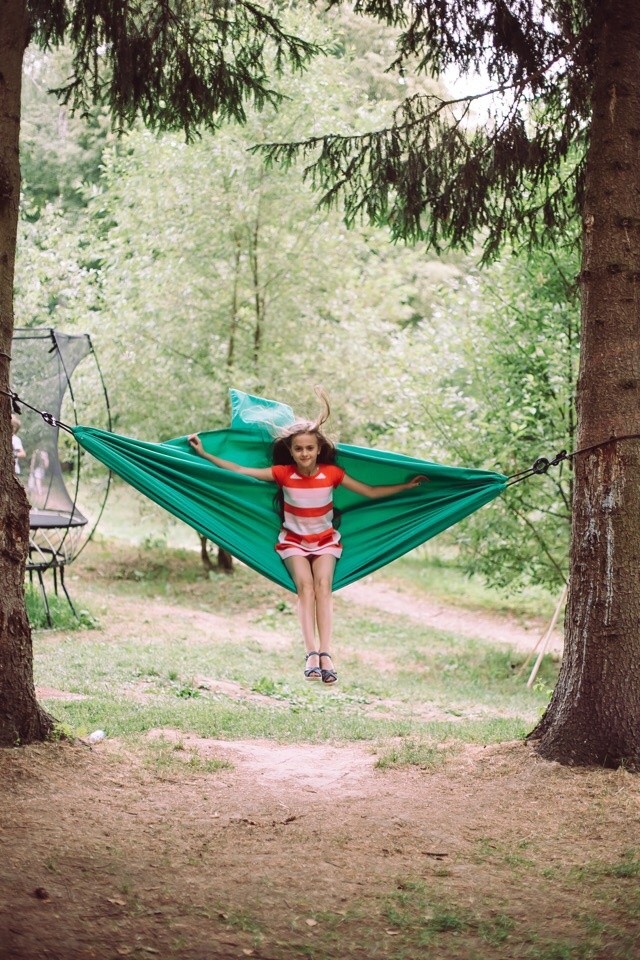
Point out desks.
[25,511,88,626]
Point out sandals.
[304,651,322,681]
[320,653,338,686]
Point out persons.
[187,387,431,684]
[11,414,27,476]
[27,449,50,512]
